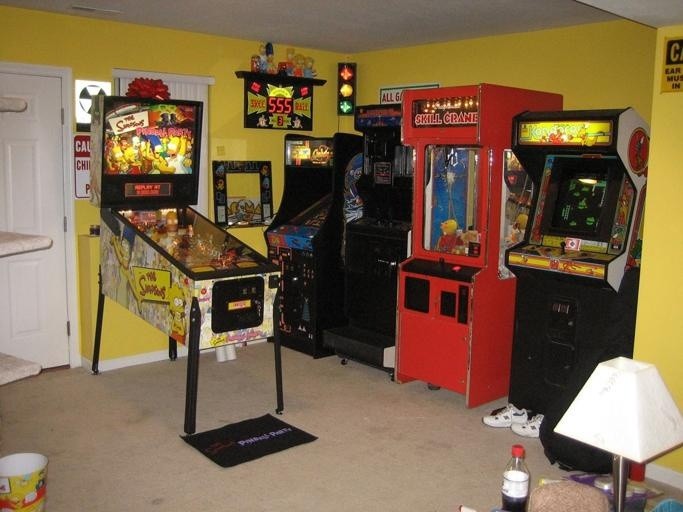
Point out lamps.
[551,355,683,511]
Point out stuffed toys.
[435,214,528,256]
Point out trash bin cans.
[0,452,48,512]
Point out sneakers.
[482,403,529,427]
[511,414,544,438]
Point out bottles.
[502,445,529,510]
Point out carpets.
[178,412,319,469]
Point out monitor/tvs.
[101,94,203,177]
[527,155,634,251]
